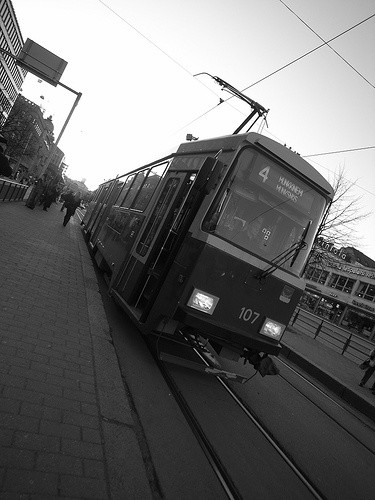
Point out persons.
[231,218,261,252]
[368,382,375,396]
[358,350,375,387]
[62,191,82,227]
[21,173,74,212]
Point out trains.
[83,133,334,383]
